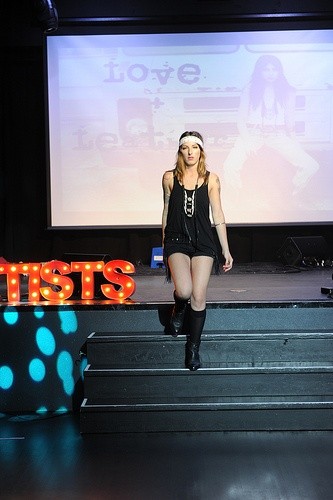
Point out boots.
[170,290,190,339]
[185,303,208,371]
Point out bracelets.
[214,222,225,226]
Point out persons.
[161,131,234,371]
[224,55,320,192]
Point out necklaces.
[180,175,199,217]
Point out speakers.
[56,253,121,297]
